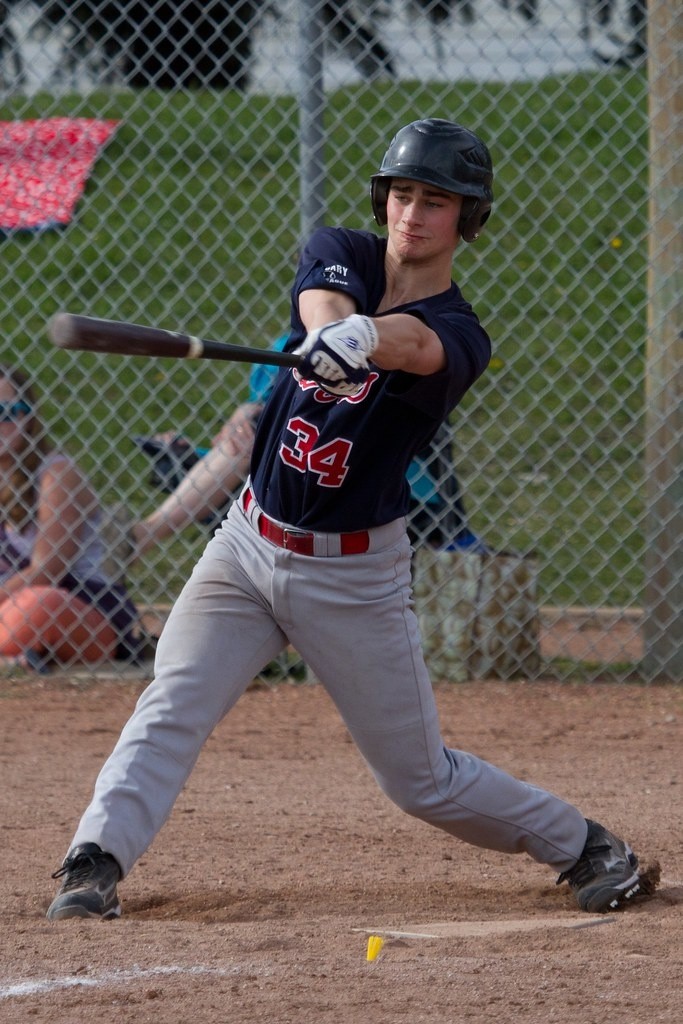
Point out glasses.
[0,402,29,422]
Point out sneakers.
[47,843,124,919]
[556,816,642,913]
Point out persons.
[42,118,662,918]
[104,242,482,676]
[0,367,151,666]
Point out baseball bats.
[46,311,303,369]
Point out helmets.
[367,117,493,243]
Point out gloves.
[293,314,377,397]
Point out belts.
[243,491,369,557]
